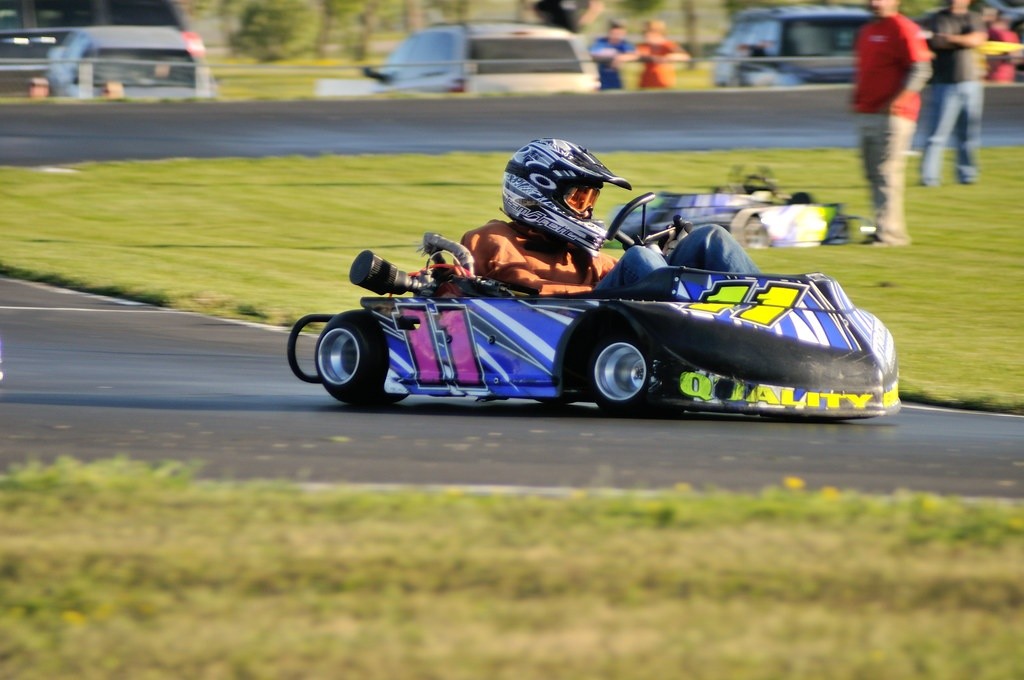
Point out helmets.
[500,138,631,259]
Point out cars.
[0,0,189,94]
[360,18,604,96]
[46,25,218,100]
[713,8,876,86]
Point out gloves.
[658,222,692,255]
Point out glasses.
[556,179,601,216]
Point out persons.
[27,78,49,98]
[532,0,604,35]
[921,1,988,184]
[637,19,692,89]
[104,81,124,99]
[588,18,641,90]
[849,0,935,246]
[460,137,764,304]
[986,18,1021,82]
